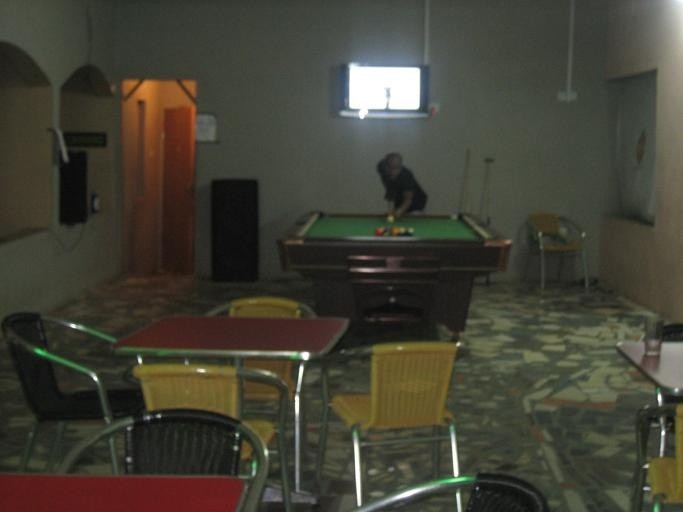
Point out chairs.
[3,311,141,477]
[522,210,588,295]
[359,474,551,511]
[184,298,318,398]
[614,323,683,512]
[321,342,465,511]
[124,363,291,512]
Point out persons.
[376,152,427,220]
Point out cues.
[387,200,392,215]
[481,158,493,287]
[459,149,473,213]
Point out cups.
[642,313,664,357]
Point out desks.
[113,315,346,493]
[3,474,247,512]
[279,207,511,334]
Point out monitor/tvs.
[338,62,429,112]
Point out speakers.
[210,179,259,281]
[60,151,88,225]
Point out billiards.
[451,215,459,221]
[375,225,414,236]
[387,215,395,222]
